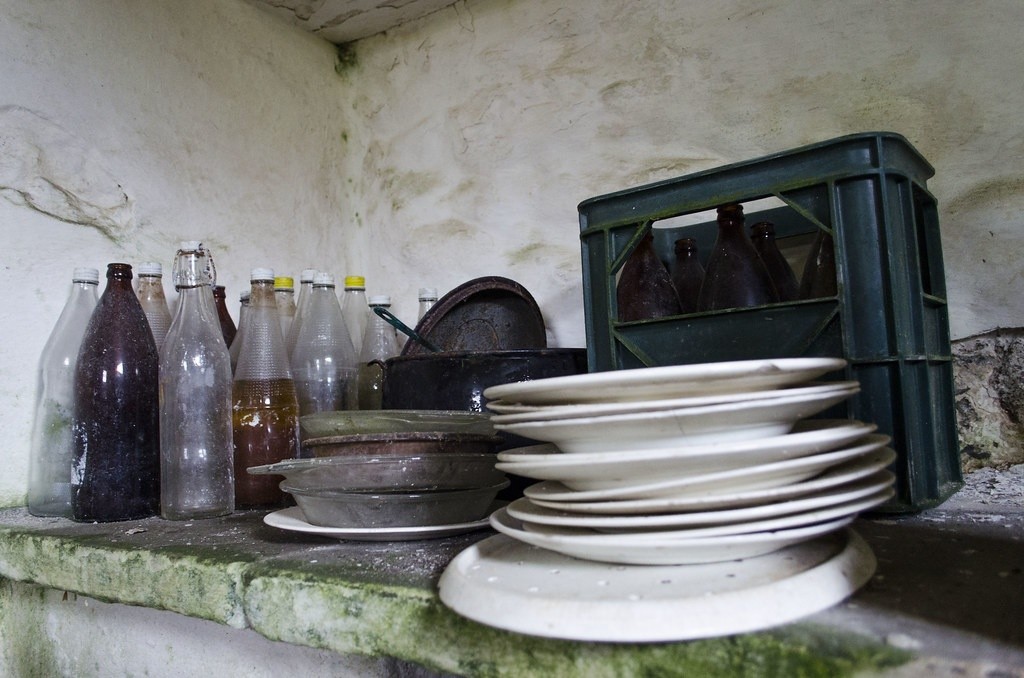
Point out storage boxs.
[579,129,966,523]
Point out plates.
[244,274,903,645]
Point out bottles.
[613,201,839,324]
[23,239,437,525]
